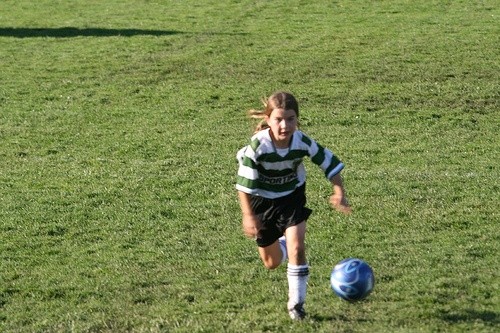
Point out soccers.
[330,259,375,301]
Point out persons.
[232,92,352,323]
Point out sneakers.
[287,304,305,321]
